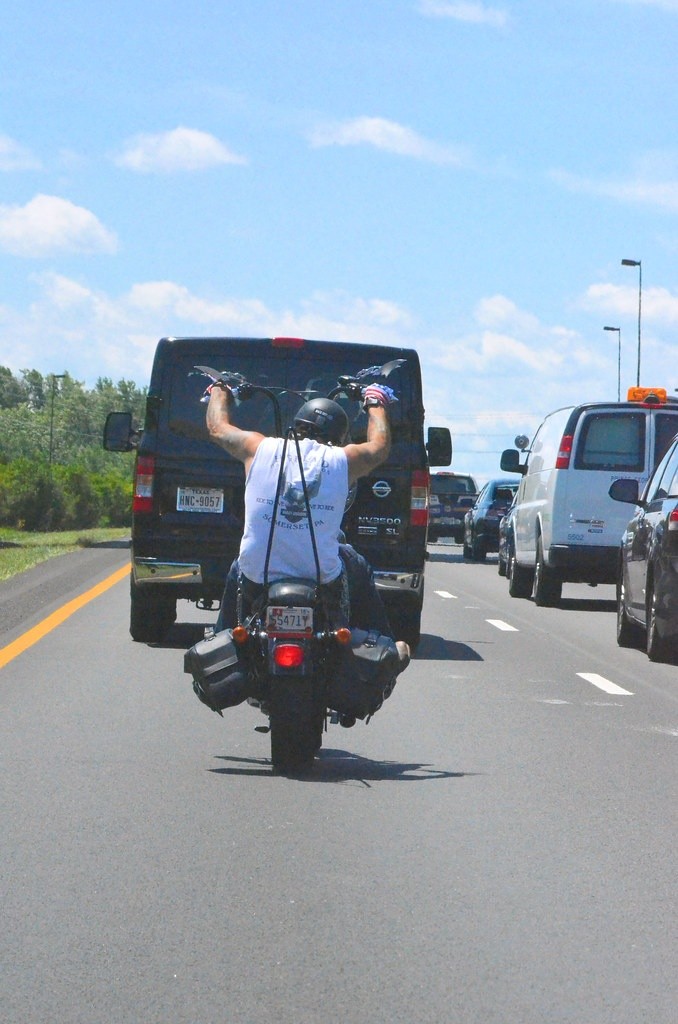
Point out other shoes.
[395,639,410,673]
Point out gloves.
[361,383,399,415]
[203,381,234,397]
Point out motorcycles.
[177,358,416,775]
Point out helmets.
[295,398,350,446]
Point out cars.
[460,477,522,579]
[604,430,677,663]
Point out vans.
[425,470,479,543]
[101,334,454,646]
[497,385,678,608]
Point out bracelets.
[362,397,381,412]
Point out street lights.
[603,326,623,402]
[47,373,67,465]
[620,257,645,386]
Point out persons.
[200,370,411,684]
